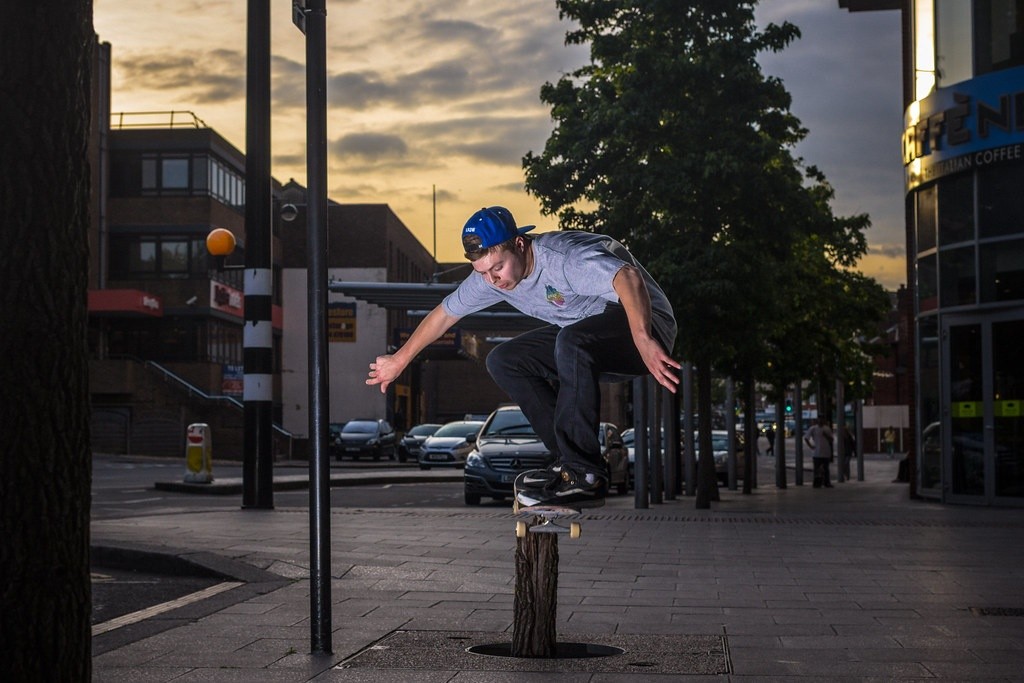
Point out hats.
[462,206,536,252]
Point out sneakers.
[518,462,610,508]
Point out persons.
[364,203,686,509]
[841,425,856,480]
[766,424,776,456]
[804,413,836,489]
[756,428,760,455]
[885,425,896,458]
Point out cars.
[334,404,751,508]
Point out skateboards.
[511,497,583,538]
